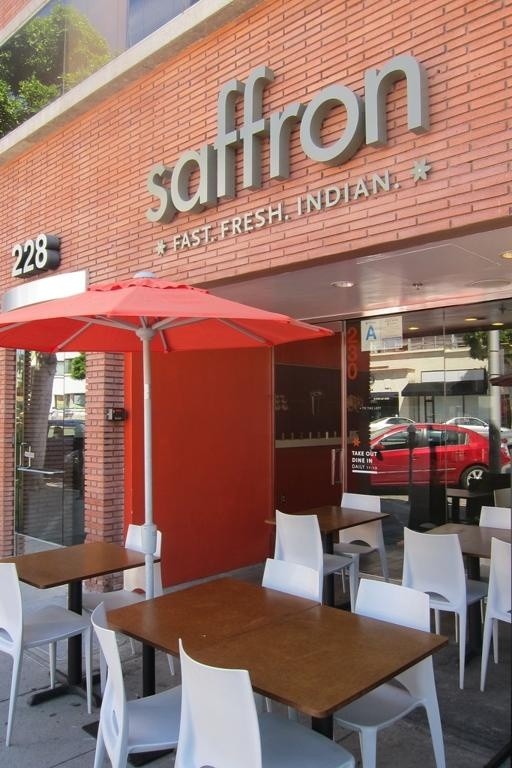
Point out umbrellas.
[1,272,339,602]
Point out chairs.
[264,488,512,691]
[254,556,320,722]
[81,524,175,695]
[173,636,357,768]
[0,561,92,746]
[333,578,449,767]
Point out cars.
[441,416,511,442]
[370,417,417,431]
[369,425,511,491]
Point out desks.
[80,578,321,767]
[2,541,163,707]
[187,605,449,741]
